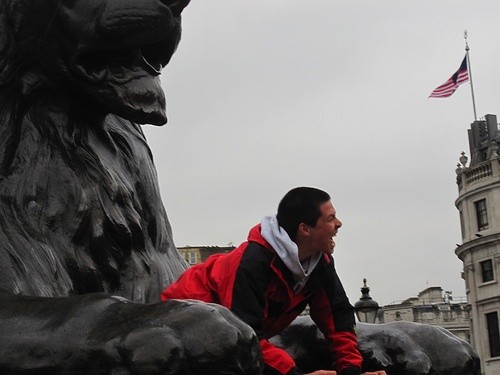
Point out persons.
[162,185,389,375]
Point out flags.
[426,52,470,100]
